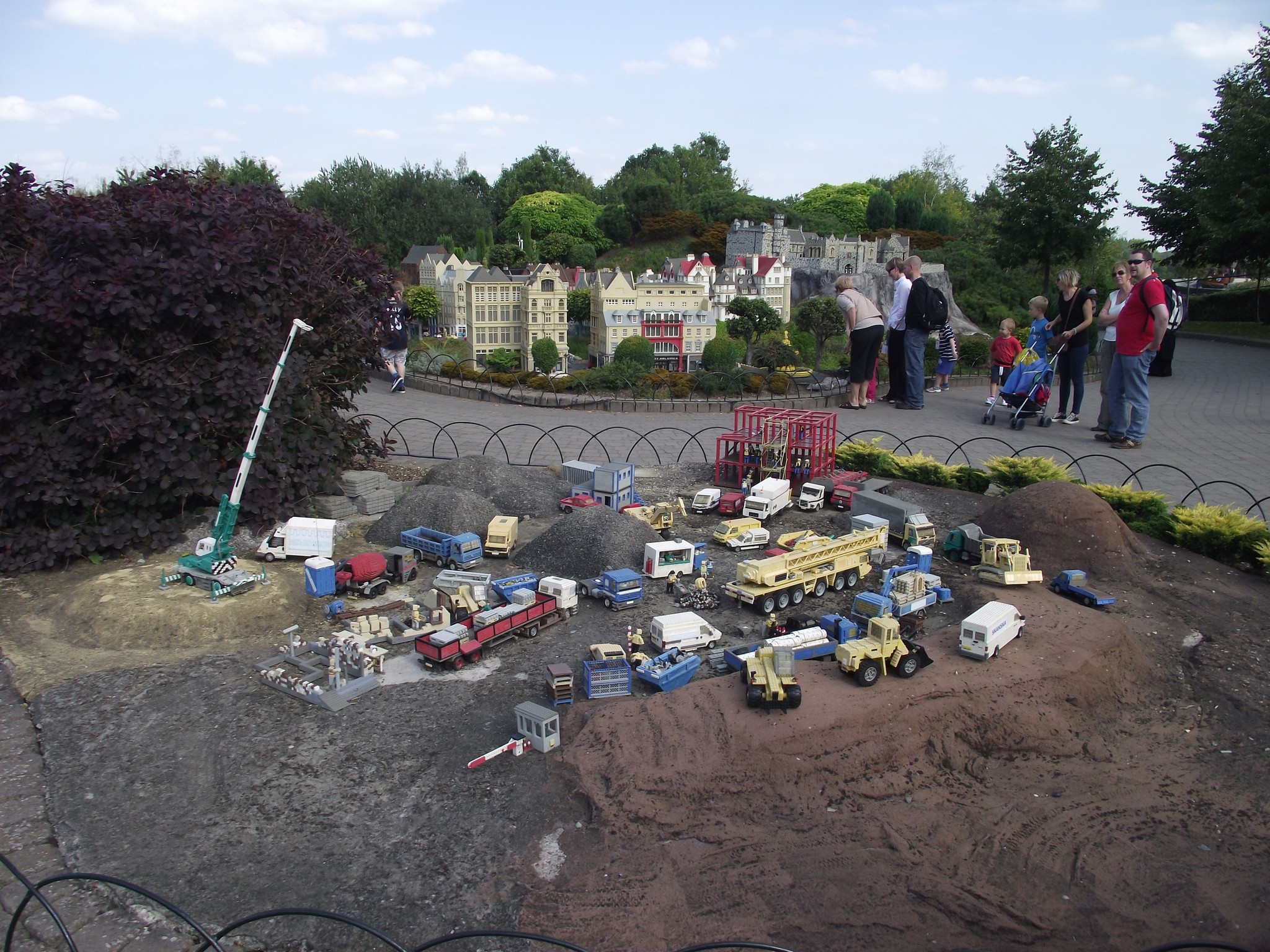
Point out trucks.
[1051,569,1117,606]
[742,477,790,526]
[484,515,519,560]
[579,568,644,612]
[850,564,937,637]
[254,515,336,561]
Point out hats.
[1088,289,1096,294]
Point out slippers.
[889,399,901,403]
[878,396,888,401]
[839,401,859,409]
[859,404,867,409]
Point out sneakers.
[985,397,996,404]
[1112,436,1142,449]
[939,384,949,390]
[1095,433,1124,442]
[1063,413,1079,424]
[926,386,941,392]
[1050,413,1067,422]
[1001,399,1007,405]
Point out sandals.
[865,398,874,404]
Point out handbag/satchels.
[1048,334,1068,351]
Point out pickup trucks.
[768,613,821,639]
[560,494,599,513]
[726,528,770,552]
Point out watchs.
[850,329,854,332]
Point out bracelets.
[1072,328,1077,336]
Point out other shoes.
[1148,369,1172,377]
[1091,427,1108,431]
[895,402,924,409]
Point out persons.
[1093,248,1168,450]
[1045,268,1093,425]
[878,256,913,405]
[1024,296,1054,413]
[1148,277,1179,378]
[984,317,1022,406]
[894,255,930,410]
[834,274,886,410]
[1090,260,1135,434]
[926,309,960,394]
[865,356,878,405]
[374,281,412,393]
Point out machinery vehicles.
[159,319,314,602]
[624,498,687,541]
[741,645,801,716]
[336,547,419,599]
[834,613,934,687]
[970,537,1043,587]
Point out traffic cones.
[777,625,786,636]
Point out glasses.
[1112,270,1126,277]
[1057,278,1060,282]
[1127,259,1148,265]
[886,267,894,274]
[835,289,838,293]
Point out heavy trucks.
[723,613,862,670]
[796,468,892,512]
[416,576,580,671]
[850,489,935,551]
[399,527,482,571]
[943,523,999,564]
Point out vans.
[692,488,721,514]
[712,517,761,545]
[958,600,1026,660]
[649,611,722,651]
[719,492,745,516]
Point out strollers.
[981,332,1069,434]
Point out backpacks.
[1139,276,1187,333]
[916,278,948,331]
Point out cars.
[423,332,467,340]
[550,371,569,378]
